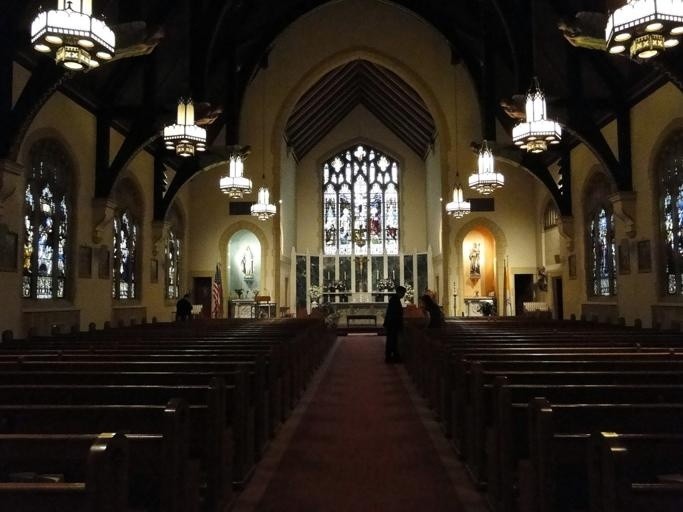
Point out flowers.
[404,285,414,301]
[376,278,396,292]
[328,280,347,290]
[305,283,324,301]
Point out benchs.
[345,315,378,327]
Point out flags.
[211,262,223,321]
[505,257,513,316]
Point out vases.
[311,299,318,310]
[383,289,389,302]
[334,289,341,302]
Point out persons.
[242,246,254,276]
[176,294,193,323]
[470,242,481,276]
[382,286,406,366]
[420,294,441,329]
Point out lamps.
[444,183,472,219]
[509,77,565,155]
[161,93,209,158]
[603,0,683,64]
[27,1,119,74]
[216,156,254,199]
[250,185,280,221]
[466,139,506,197]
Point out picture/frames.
[78,243,93,280]
[618,242,632,276]
[636,240,653,274]
[150,258,159,284]
[567,254,577,281]
[0,223,19,274]
[97,244,111,279]
